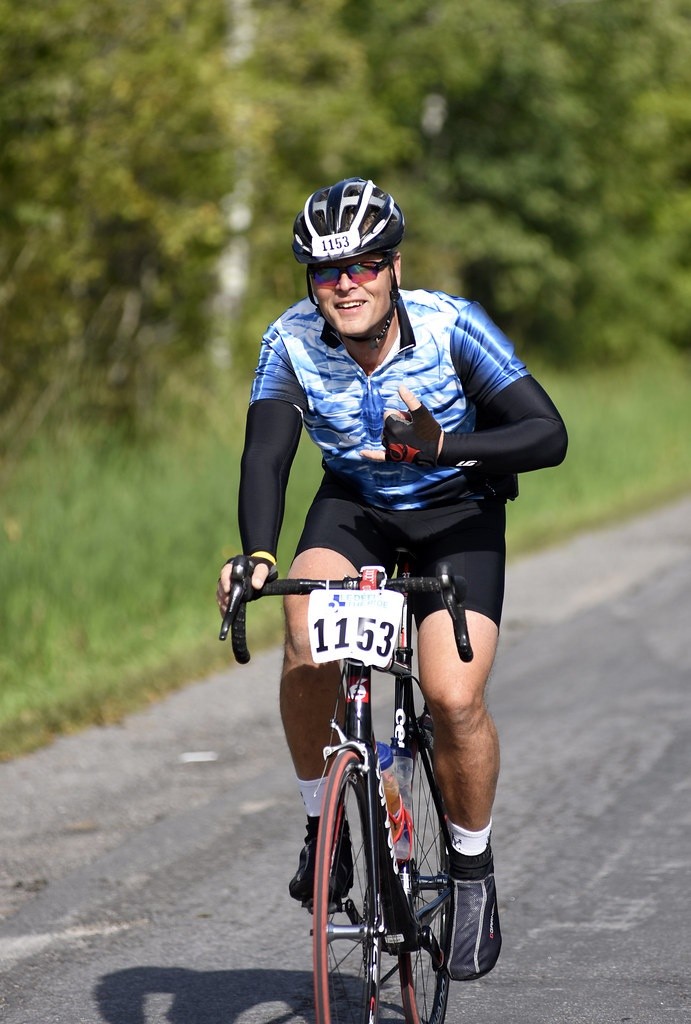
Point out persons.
[213,175,570,981]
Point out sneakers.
[444,846,502,980]
[288,815,354,902]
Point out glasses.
[307,257,389,287]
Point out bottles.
[375,742,403,839]
[390,737,415,860]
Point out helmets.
[292,177,406,263]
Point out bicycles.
[219,555,474,1024]
